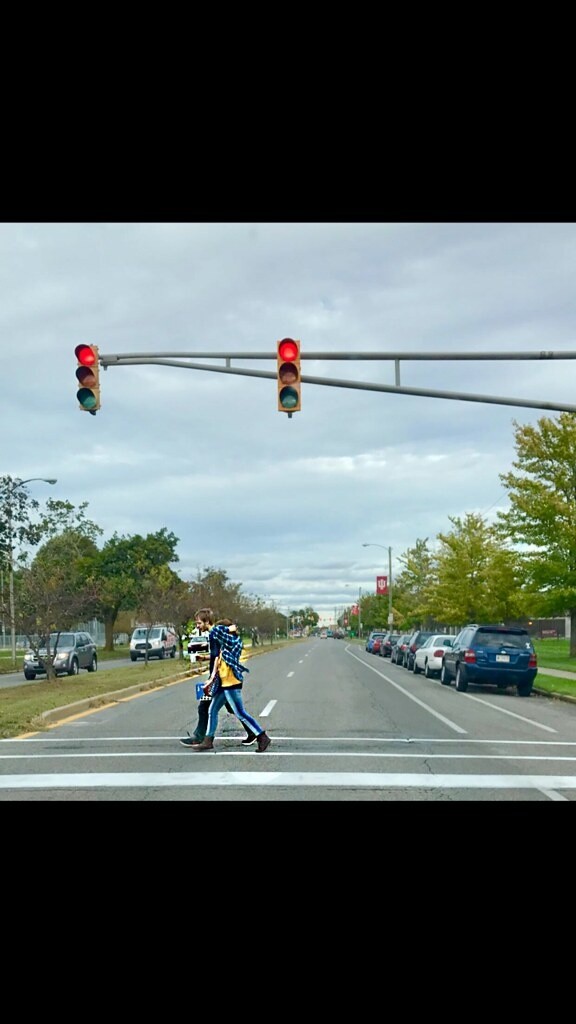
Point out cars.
[366,631,386,652]
[379,635,403,657]
[390,634,414,665]
[187,636,210,654]
[371,635,384,654]
[414,635,456,678]
[320,629,345,640]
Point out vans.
[129,627,177,661]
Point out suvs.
[23,632,98,681]
[402,631,445,670]
[440,623,539,696]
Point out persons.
[193,619,271,752]
[180,608,257,747]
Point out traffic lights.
[73,343,103,412]
[277,337,306,413]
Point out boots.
[193,736,215,750]
[254,730,271,752]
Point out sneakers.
[241,734,256,746]
[180,737,201,747]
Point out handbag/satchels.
[204,673,221,697]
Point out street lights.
[362,543,394,635]
[345,584,361,639]
[8,476,59,666]
